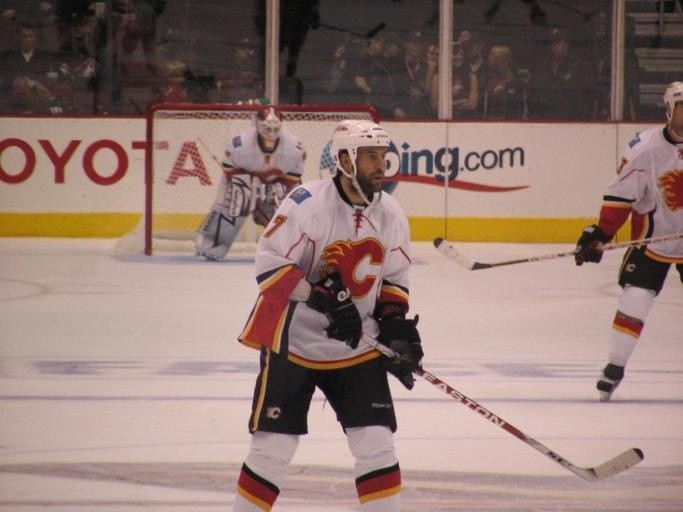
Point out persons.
[573,81,681,392]
[230,116,426,512]
[194,106,310,263]
[0,1,670,122]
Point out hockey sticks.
[360,331,644,484]
[430,233,683,269]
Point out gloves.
[309,271,363,348]
[379,315,424,391]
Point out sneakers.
[598,364,625,395]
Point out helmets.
[663,80,682,121]
[256,107,283,142]
[329,119,390,178]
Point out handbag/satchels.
[574,224,611,267]
